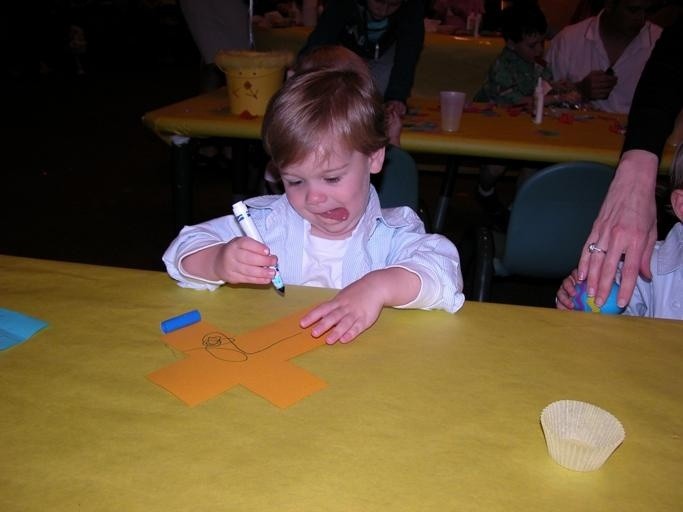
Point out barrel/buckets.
[215,48,294,119]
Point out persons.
[576,4,683,310]
[2,0,663,118]
[160,66,466,346]
[554,141,683,321]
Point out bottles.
[467,13,479,39]
[532,77,544,125]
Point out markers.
[232,201,285,294]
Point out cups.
[537,400,624,468]
[438,91,465,132]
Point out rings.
[588,243,606,255]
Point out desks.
[138,84,683,243]
[0,252,682,512]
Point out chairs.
[465,160,616,303]
[369,145,419,212]
